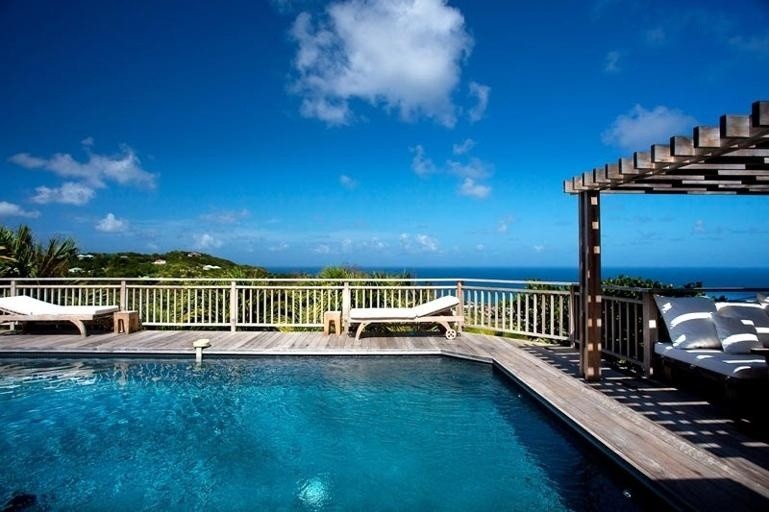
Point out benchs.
[652,338,769,400]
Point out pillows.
[710,313,763,354]
[713,300,769,348]
[755,292,769,316]
[652,294,724,350]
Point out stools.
[323,311,343,335]
[113,310,139,336]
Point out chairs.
[0,294,120,338]
[349,294,465,341]
